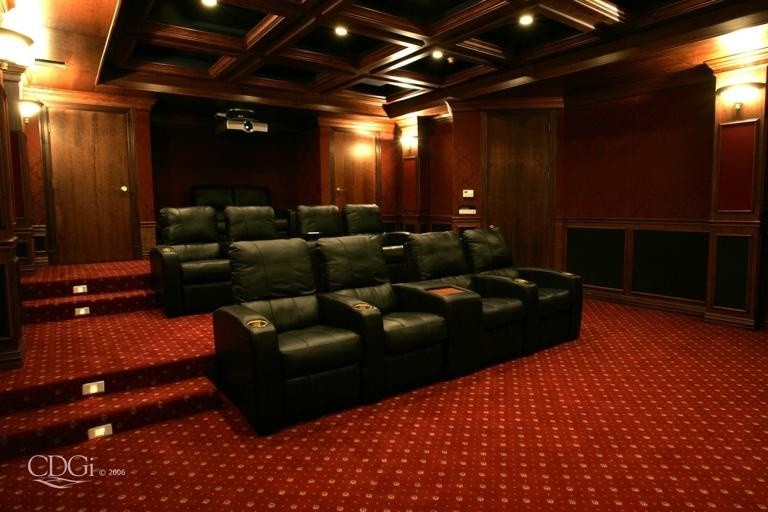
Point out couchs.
[149,200,586,439]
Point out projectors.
[224,108,269,135]
